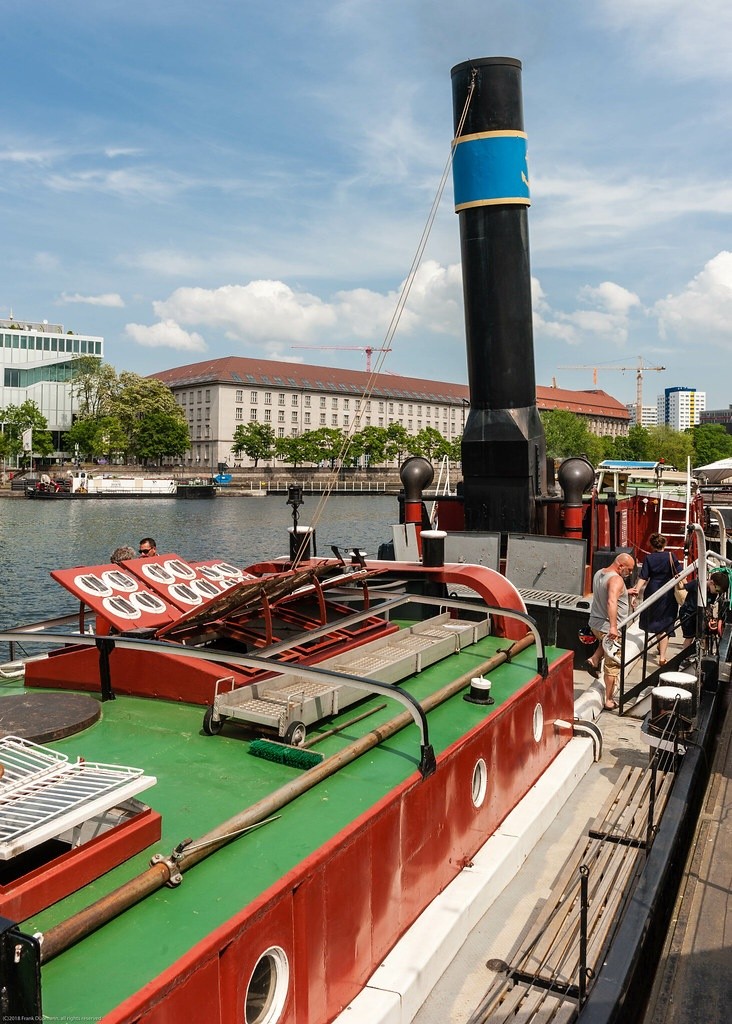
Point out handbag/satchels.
[675,578,688,605]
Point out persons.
[139,539,159,558]
[633,533,682,666]
[110,546,134,568]
[37,480,60,492]
[679,572,729,649]
[234,462,236,467]
[585,553,637,711]
[239,463,241,467]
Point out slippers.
[586,660,602,680]
[605,702,620,710]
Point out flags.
[22,428,32,451]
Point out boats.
[376,55,732,671]
[0,455,604,1024]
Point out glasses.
[713,584,721,593]
[138,547,154,554]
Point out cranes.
[289,344,392,373]
[554,354,666,427]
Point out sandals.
[657,644,661,655]
[660,658,666,667]
